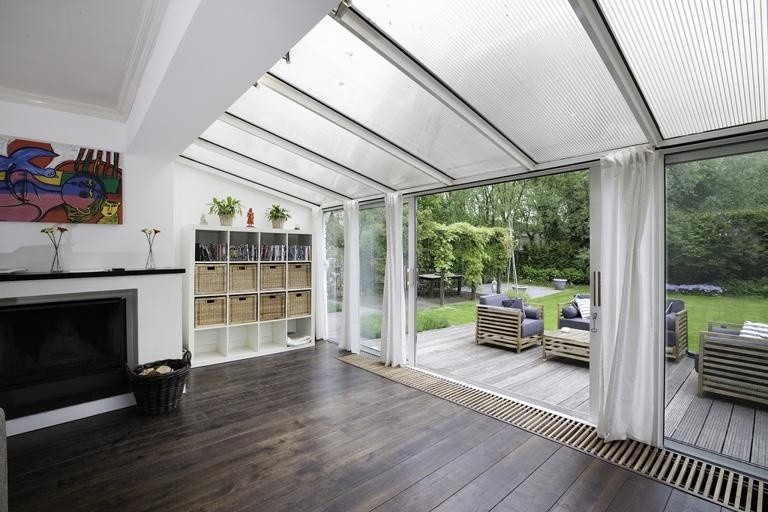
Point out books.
[196,242,312,261]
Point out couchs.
[556,293,689,363]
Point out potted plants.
[205,193,244,225]
[264,201,291,229]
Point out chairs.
[694,318,767,408]
[474,292,545,354]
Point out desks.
[418,273,463,300]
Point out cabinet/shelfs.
[182,224,316,369]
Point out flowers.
[39,223,71,273]
[139,227,161,270]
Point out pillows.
[738,319,767,341]
[501,298,527,318]
[576,298,591,321]
[562,305,579,318]
[524,304,541,319]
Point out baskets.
[126,349,192,416]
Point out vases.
[552,278,568,290]
[48,249,65,273]
[143,247,158,270]
[510,284,527,293]
